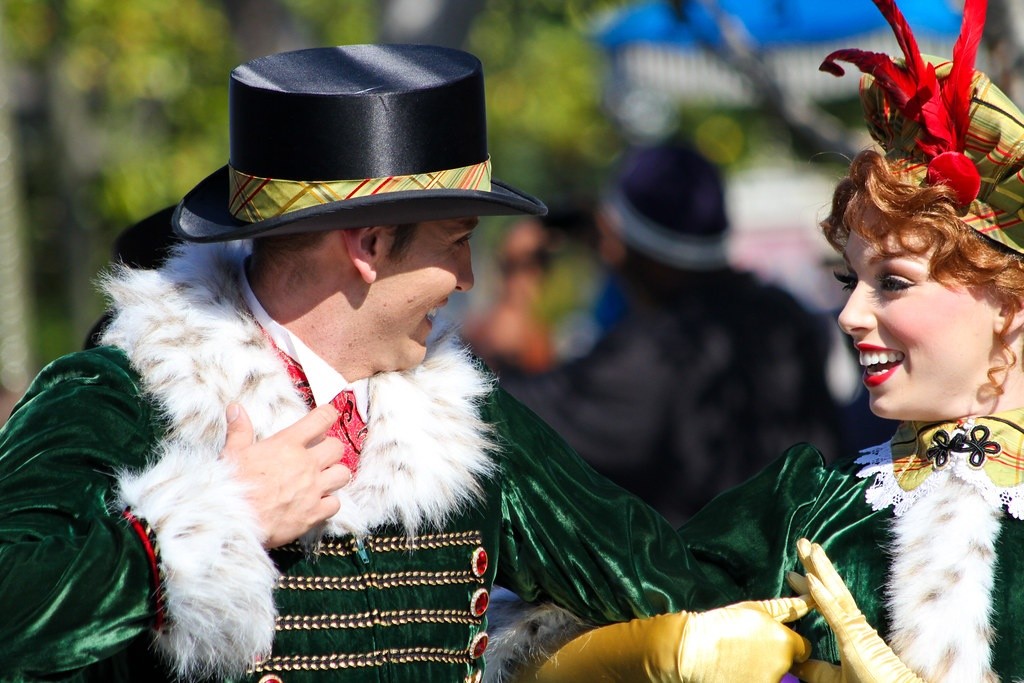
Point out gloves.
[785,537,927,683]
[500,594,814,683]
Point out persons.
[0,46,749,683]
[483,53,1024,683]
[461,136,828,529]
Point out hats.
[595,142,732,272]
[172,42,548,245]
[819,0,1024,256]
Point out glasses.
[496,247,554,278]
